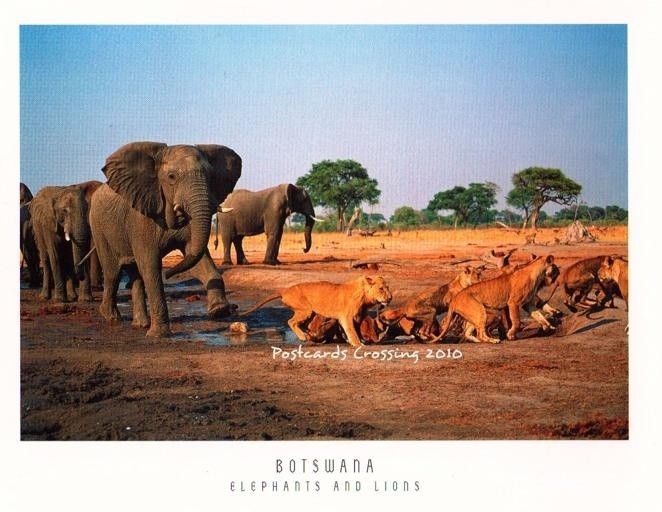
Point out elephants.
[87,139,244,338]
[213,182,325,267]
[21,180,106,304]
